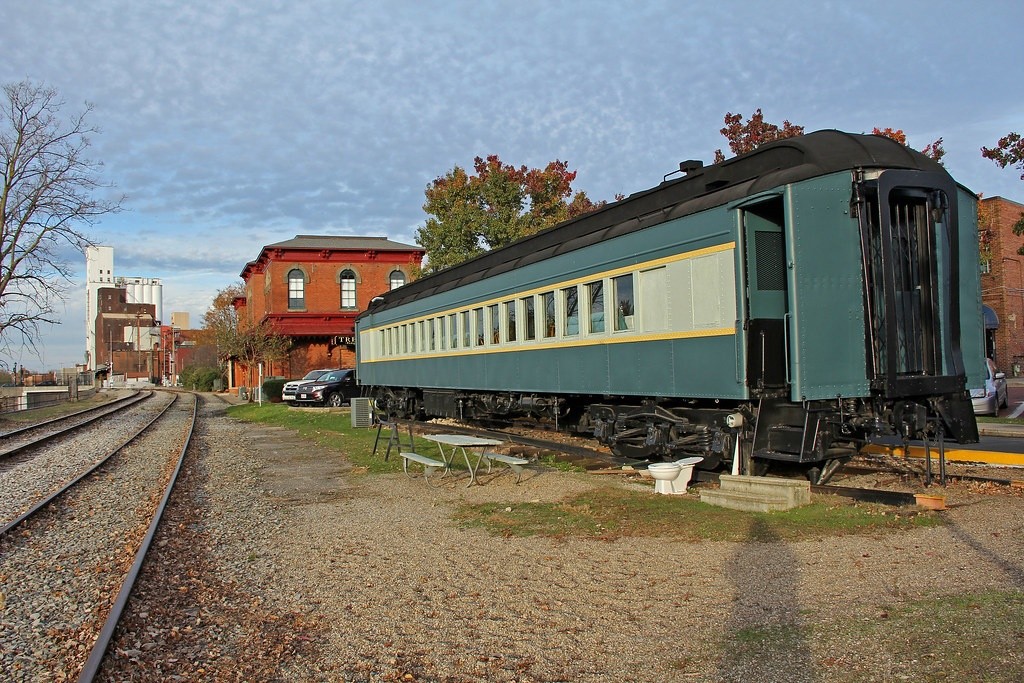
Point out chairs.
[372,394,415,463]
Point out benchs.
[399,434,528,489]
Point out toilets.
[647,456,704,496]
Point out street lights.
[13,362,18,387]
[20,364,24,387]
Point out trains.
[347,128,998,513]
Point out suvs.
[282,369,334,408]
[295,368,380,408]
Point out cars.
[968,357,1011,420]
